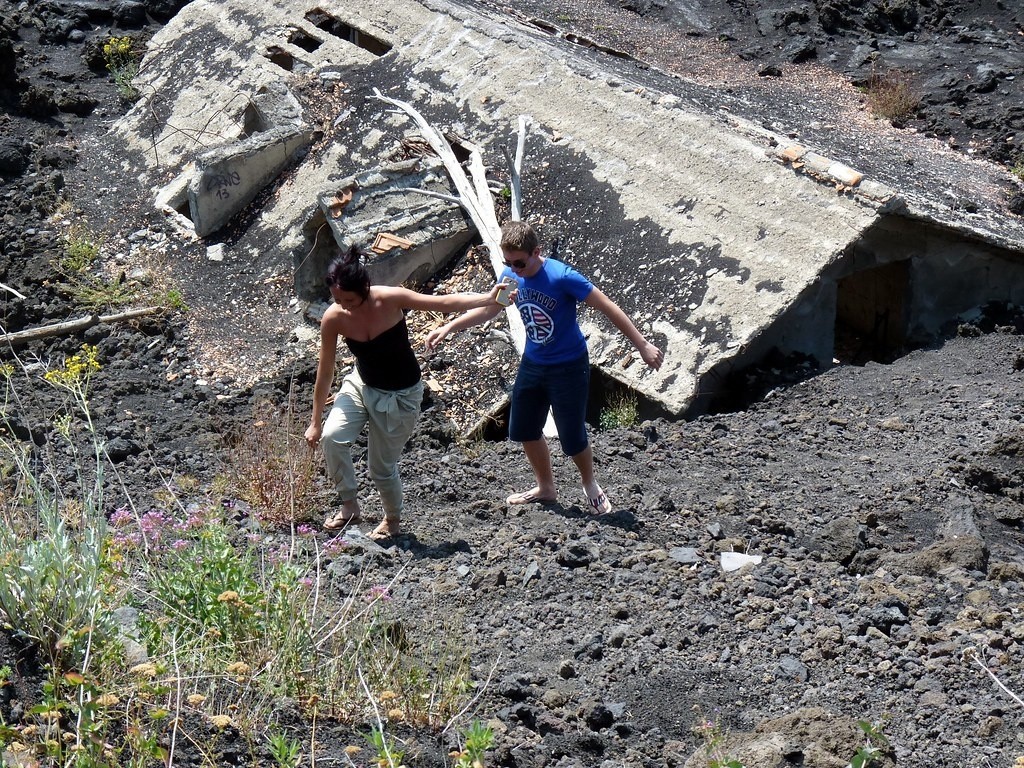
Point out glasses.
[502,251,532,268]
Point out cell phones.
[496,276,518,307]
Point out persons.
[305,242,520,540]
[425,222,664,516]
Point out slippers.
[506,491,557,504]
[582,480,612,517]
[322,515,362,530]
[365,529,401,542]
